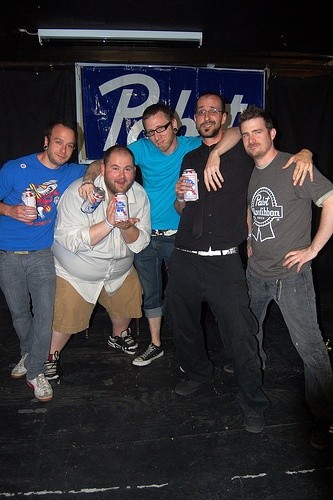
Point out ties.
[192,148,211,240]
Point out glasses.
[144,121,171,137]
[195,108,223,115]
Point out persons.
[44,145,151,387]
[224,104,333,448]
[0,118,89,402]
[78,103,242,366]
[162,94,314,433]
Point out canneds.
[114,192,129,222]
[21,188,37,217]
[81,186,105,214]
[182,168,199,202]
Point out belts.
[151,230,178,236]
[0,250,37,255]
[174,247,239,256]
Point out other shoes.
[175,376,214,395]
[246,415,265,433]
[310,416,333,448]
[223,361,265,373]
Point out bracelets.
[177,198,184,202]
[105,220,113,228]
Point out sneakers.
[26,373,53,402]
[11,353,28,379]
[44,351,60,386]
[132,343,165,366]
[108,328,138,355]
